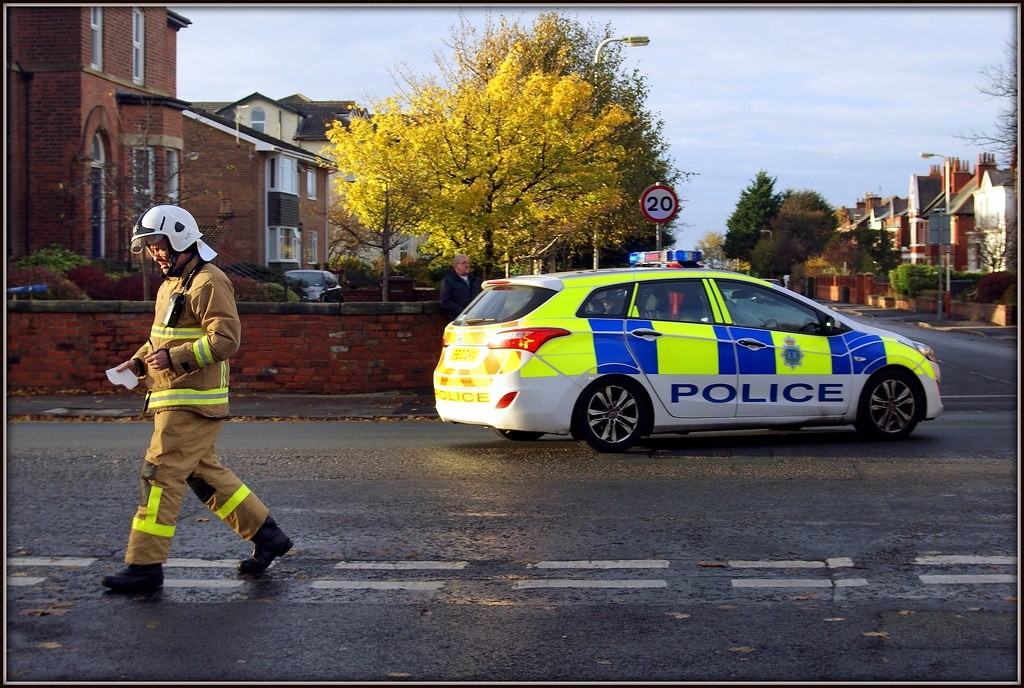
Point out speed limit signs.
[639,184,679,223]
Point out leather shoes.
[237,515,293,574]
[101,563,164,591]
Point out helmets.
[131,203,203,252]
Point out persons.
[101,204,295,594]
[440,254,481,322]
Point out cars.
[758,279,788,302]
[723,290,760,310]
[280,269,342,301]
[432,251,947,451]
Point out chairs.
[644,289,669,320]
[679,293,704,323]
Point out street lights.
[759,230,773,240]
[919,151,950,320]
[593,35,651,269]
